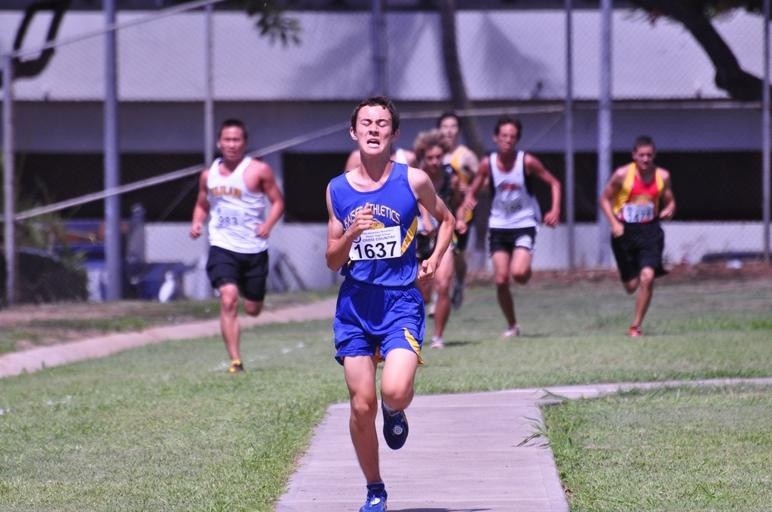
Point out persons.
[191,118,285,373]
[465,115,561,337]
[434,111,488,307]
[601,135,677,336]
[325,97,456,512]
[406,129,461,347]
[344,148,418,170]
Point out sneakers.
[504,324,521,337]
[451,289,463,308]
[428,304,436,318]
[381,399,409,450]
[229,363,243,372]
[359,483,388,512]
[629,326,641,337]
[430,337,443,350]
